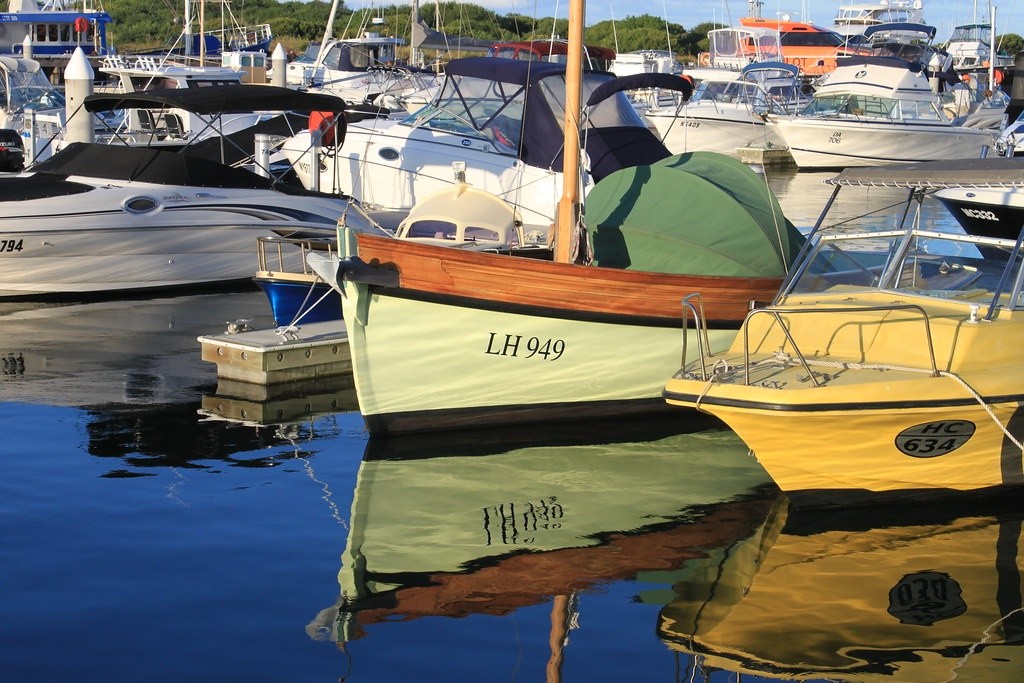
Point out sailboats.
[0,0,684,181]
[657,487,1024,683]
[306,1,986,435]
[303,434,786,683]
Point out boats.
[936,0,1016,74]
[763,23,1010,168]
[1,294,333,466]
[256,181,554,326]
[644,62,815,162]
[0,84,394,302]
[932,154,1024,260]
[659,158,1024,491]
[699,0,896,82]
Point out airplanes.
[825,0,926,49]
